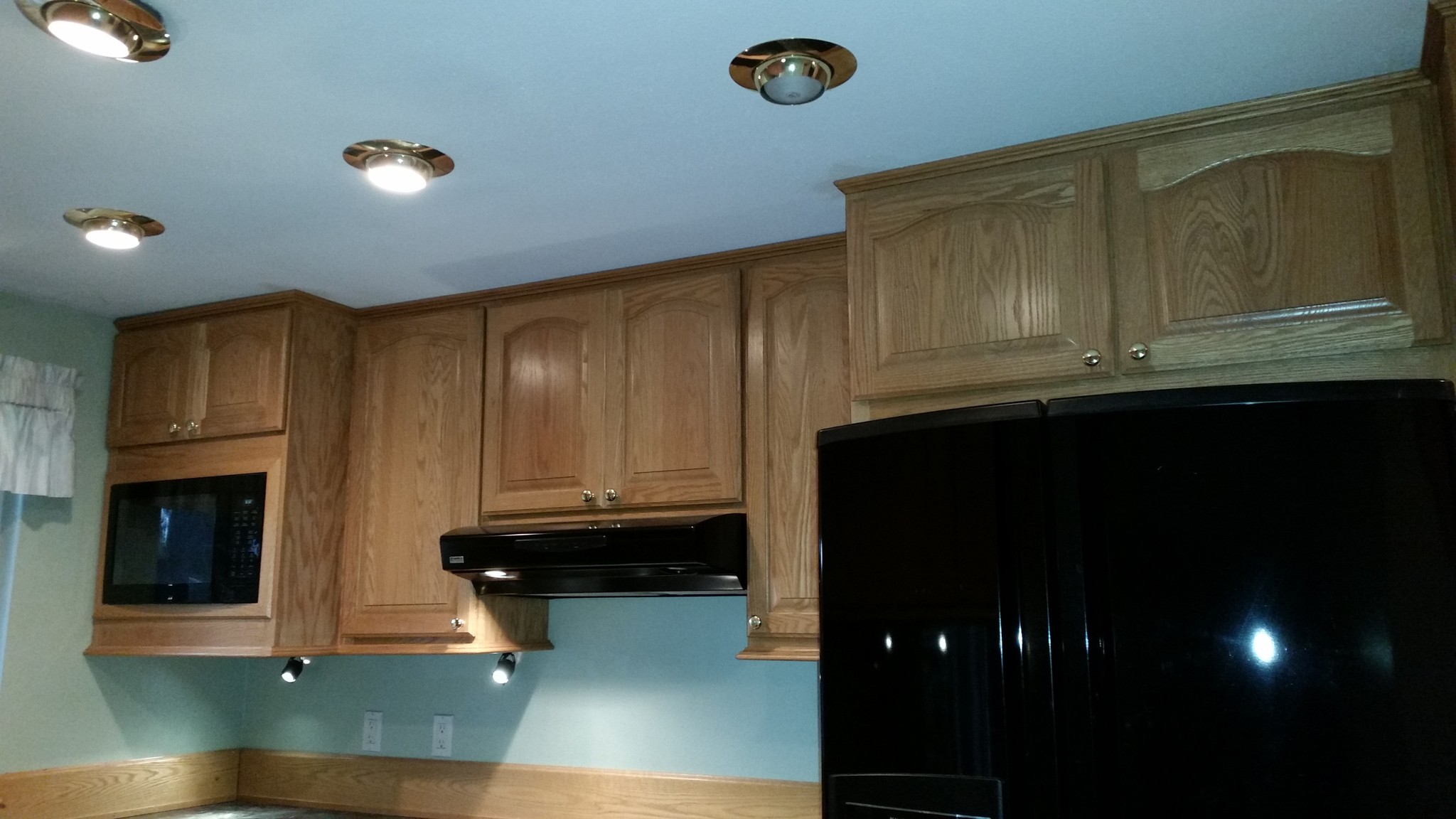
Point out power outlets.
[361,711,383,752]
[432,714,453,757]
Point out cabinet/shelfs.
[734,232,866,664]
[484,243,748,527]
[80,281,339,656]
[332,296,554,652]
[822,61,1456,411]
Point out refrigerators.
[815,376,1456,818]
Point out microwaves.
[104,469,267,604]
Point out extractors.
[437,514,747,597]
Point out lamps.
[13,0,174,64]
[730,36,859,111]
[280,655,307,683]
[491,652,516,684]
[61,207,165,250]
[342,138,455,195]
